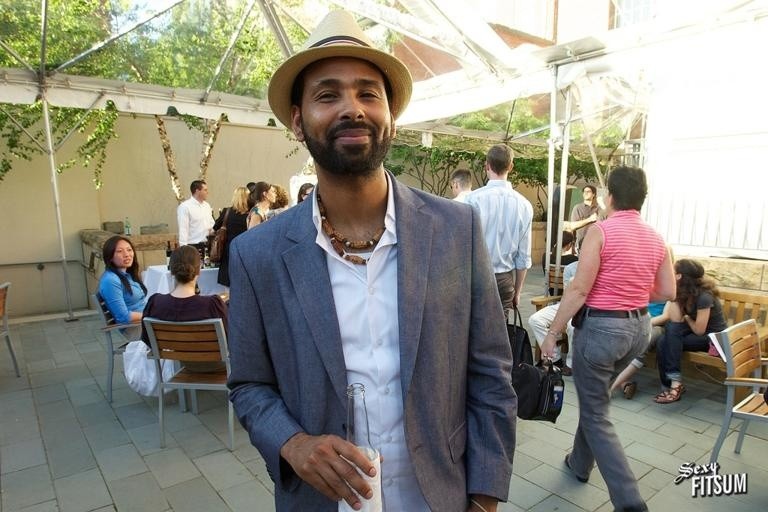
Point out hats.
[269,10,412,131]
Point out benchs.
[530,289,768,396]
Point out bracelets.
[470,497,489,511]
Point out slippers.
[623,382,636,399]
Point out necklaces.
[329,221,385,249]
[315,192,370,267]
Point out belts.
[586,307,647,318]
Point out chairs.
[92,292,188,412]
[0,281,21,377]
[143,317,236,452]
[546,264,566,290]
[709,319,768,464]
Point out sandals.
[653,384,686,403]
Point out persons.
[175,178,316,287]
[447,169,475,196]
[139,244,229,374]
[229,57,518,512]
[527,184,729,406]
[448,143,536,327]
[540,165,679,512]
[98,236,150,341]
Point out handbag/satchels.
[506,305,533,365]
[210,227,226,262]
[122,340,180,396]
[511,355,564,424]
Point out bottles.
[124,216,131,235]
[338,380,384,507]
[165,240,173,270]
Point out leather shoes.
[614,504,648,512]
[566,454,588,482]
